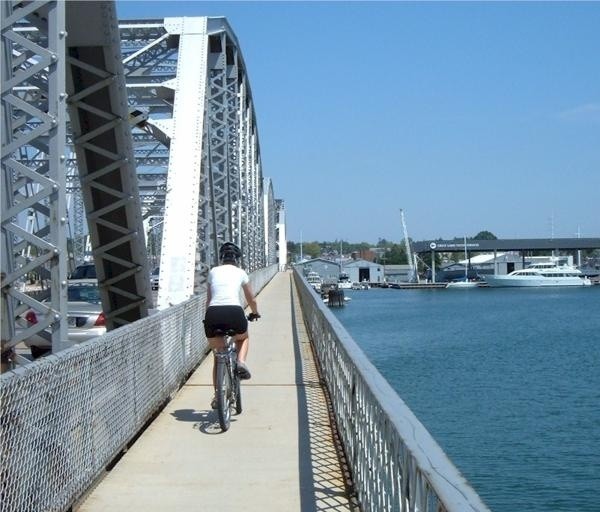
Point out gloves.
[486,262,591,287]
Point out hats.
[149,266,159,290]
[26,260,107,358]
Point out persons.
[203,241,262,409]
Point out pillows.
[218,242,242,259]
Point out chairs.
[233,362,251,380]
[210,396,222,409]
